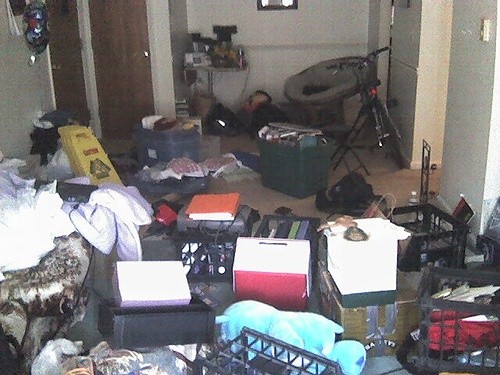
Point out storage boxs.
[384,203,470,272]
[187,326,345,375]
[322,219,398,308]
[257,141,329,199]
[130,128,200,166]
[163,208,252,282]
[234,141,259,173]
[234,215,320,313]
[110,260,191,307]
[413,267,500,374]
[317,259,418,358]
[98,299,214,349]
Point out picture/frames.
[257,0,298,10]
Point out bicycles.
[325,46,406,170]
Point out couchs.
[0,179,99,375]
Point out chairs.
[284,55,369,128]
[318,93,375,178]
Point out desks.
[185,65,247,96]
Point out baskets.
[383,201,473,273]
[413,269,500,375]
[192,326,345,375]
[178,231,239,283]
